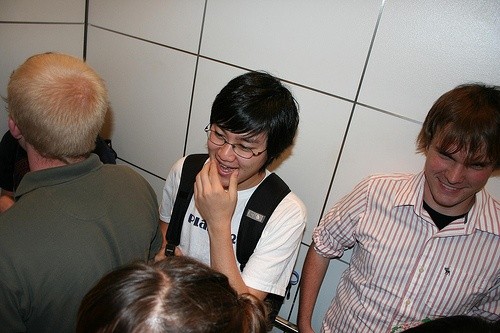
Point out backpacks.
[165,153,299,331]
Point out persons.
[0,53,162,333]
[297,82,500,333]
[400,315,500,333]
[0,129,117,213]
[154,71,307,303]
[76,256,270,333]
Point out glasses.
[204,123,267,159]
